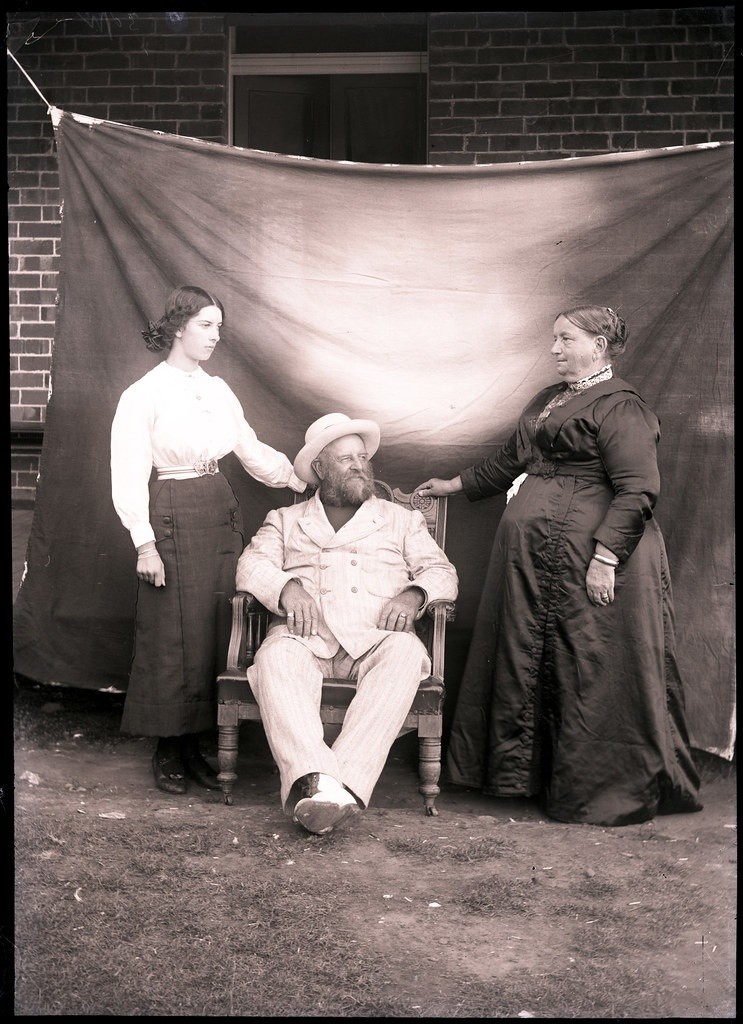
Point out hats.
[292,413,381,487]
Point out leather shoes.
[152,749,221,794]
[292,772,362,835]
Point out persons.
[414,304,704,826]
[110,284,316,795]
[233,413,459,836]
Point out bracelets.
[137,548,159,560]
[592,553,618,567]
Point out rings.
[287,613,293,617]
[400,613,407,618]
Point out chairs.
[217,486,458,817]
